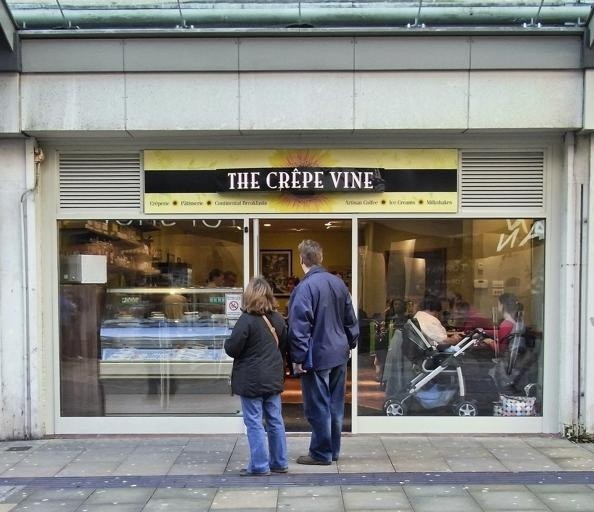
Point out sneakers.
[240,467,288,476]
[298,456,332,465]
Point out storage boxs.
[67,251,109,285]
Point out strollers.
[383,311,491,416]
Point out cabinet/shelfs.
[97,286,247,415]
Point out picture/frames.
[258,249,293,299]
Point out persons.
[288,239,359,465]
[202,268,301,325]
[223,277,288,478]
[331,271,527,392]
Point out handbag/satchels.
[493,393,536,416]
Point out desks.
[60,284,108,416]
